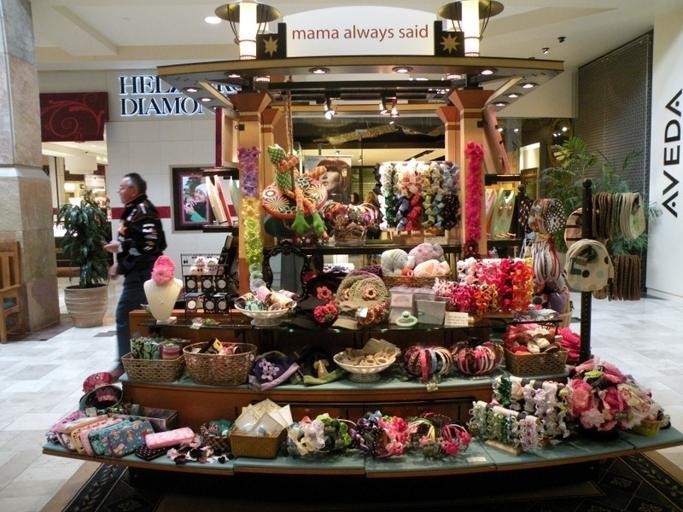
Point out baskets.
[557,309,575,329]
[198,420,232,458]
[181,339,258,388]
[118,351,185,386]
[229,425,284,459]
[501,342,571,379]
[634,410,667,440]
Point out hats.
[254,349,301,394]
[298,339,350,385]
[77,371,125,413]
[564,208,585,246]
[332,301,365,332]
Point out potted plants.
[535,132,656,300]
[58,186,112,328]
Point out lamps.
[437,0,501,56]
[215,0,280,60]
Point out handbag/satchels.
[592,192,646,243]
[561,240,616,296]
[510,194,532,236]
[527,196,569,237]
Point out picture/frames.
[170,165,207,230]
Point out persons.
[184,183,208,225]
[312,159,352,204]
[102,172,167,383]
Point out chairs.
[0,241,29,344]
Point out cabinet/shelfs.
[261,233,533,303]
[42,308,683,489]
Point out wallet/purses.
[87,419,132,456]
[52,413,105,452]
[135,443,174,461]
[108,420,156,459]
[78,418,131,458]
[70,418,117,462]
[104,420,148,459]
[142,425,196,451]
